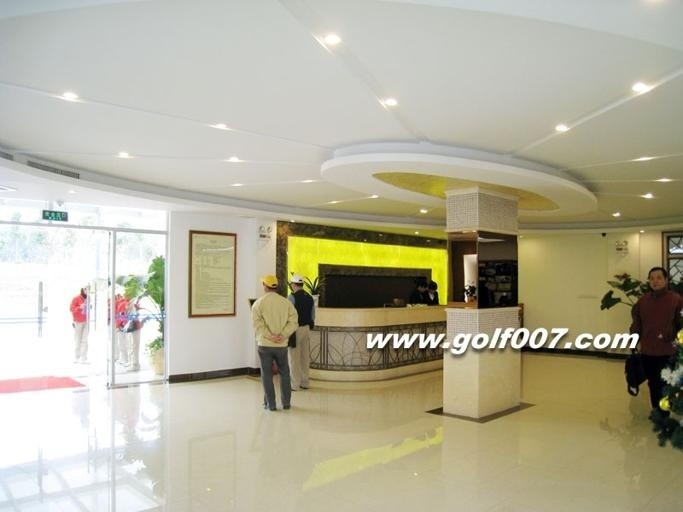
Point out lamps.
[258,225,271,233]
[614,240,627,248]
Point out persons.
[629,267,682,417]
[408,280,427,304]
[285,275,315,391]
[424,281,439,305]
[69,282,141,371]
[250,274,299,411]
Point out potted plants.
[303,275,326,309]
[115,255,164,378]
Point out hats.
[291,275,304,284]
[262,275,278,289]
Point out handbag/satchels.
[123,320,137,333]
[625,350,648,387]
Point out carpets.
[0,374,85,393]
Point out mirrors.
[444,229,518,308]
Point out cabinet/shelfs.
[478,259,517,306]
